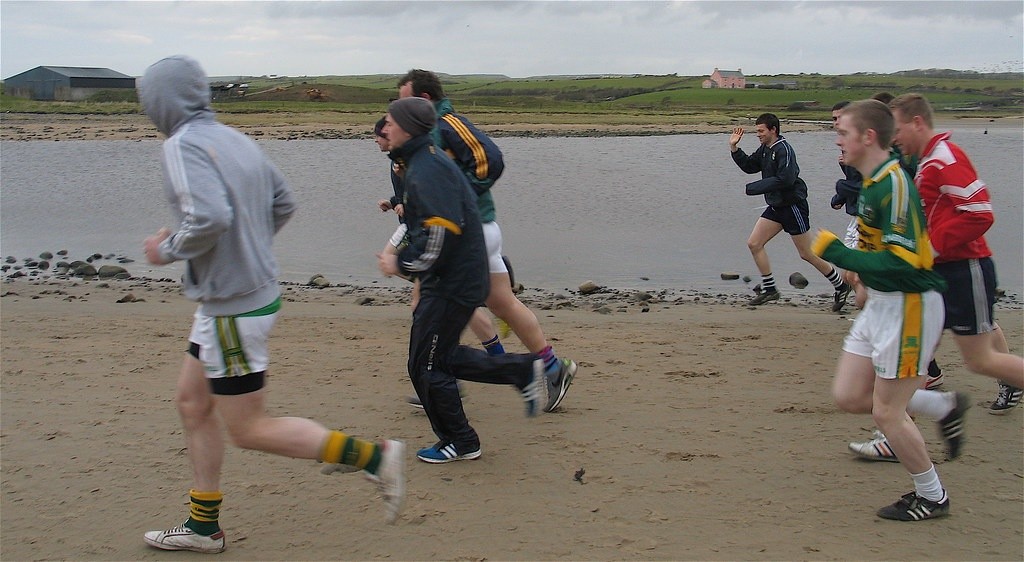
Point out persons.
[373,71,579,464]
[728,113,852,313]
[809,92,1024,521]
[132,55,407,554]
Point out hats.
[374,116,387,138]
[389,97,436,137]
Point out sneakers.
[144,520,226,553]
[877,488,950,521]
[543,357,578,412]
[848,430,899,462]
[748,289,779,306]
[516,353,548,417]
[989,379,1024,415]
[373,439,404,525]
[925,369,944,389]
[931,391,972,460]
[405,395,424,408]
[833,284,851,311]
[416,439,481,463]
